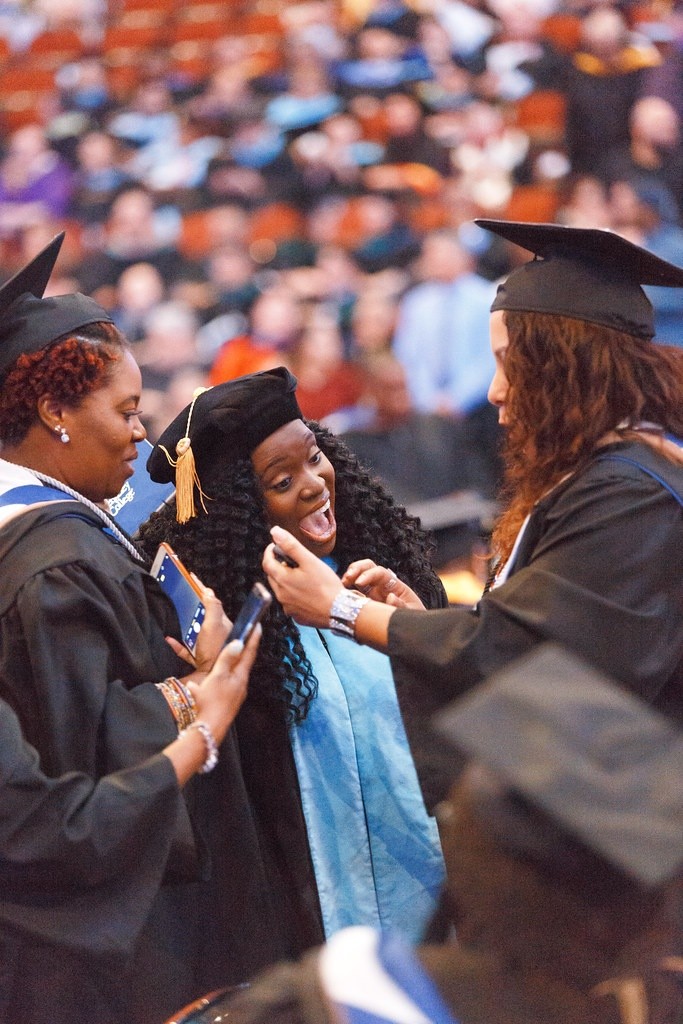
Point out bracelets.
[326,589,370,642]
[157,674,220,775]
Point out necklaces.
[0,459,152,569]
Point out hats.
[474,218,683,339]
[432,638,683,927]
[0,230,115,369]
[146,366,302,524]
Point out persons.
[262,220,683,811]
[0,234,310,1024]
[0,621,263,958]
[168,646,683,1024]
[0,0,683,611]
[140,365,453,943]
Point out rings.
[361,583,375,592]
[383,576,397,588]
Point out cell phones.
[147,542,210,657]
[271,544,299,569]
[222,581,273,653]
